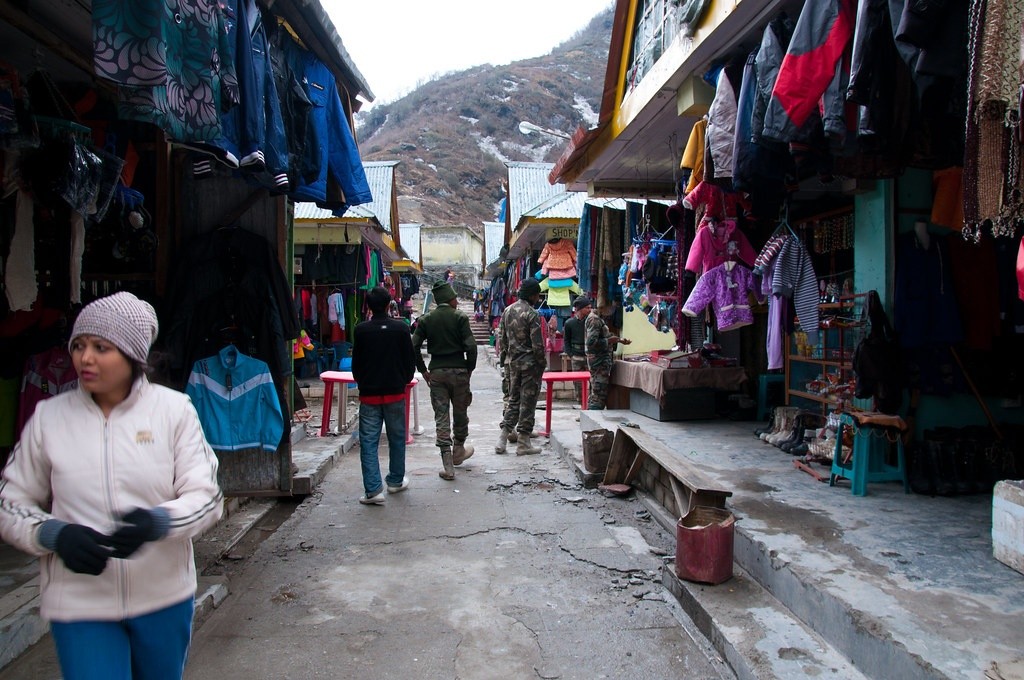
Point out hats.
[68,291,159,369]
[573,296,590,311]
[520,277,541,295]
[431,281,457,305]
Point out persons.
[351,287,415,505]
[495,277,547,456]
[0,290,224,680]
[585,289,633,411]
[564,295,592,410]
[413,281,478,480]
[444,265,454,288]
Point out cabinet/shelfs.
[784,290,877,426]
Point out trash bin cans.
[582,429,614,473]
[676,506,735,585]
[338,357,357,388]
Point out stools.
[829,411,911,498]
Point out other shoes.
[530,432,539,438]
[359,493,384,503]
[507,430,517,442]
[387,478,409,493]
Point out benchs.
[602,425,732,540]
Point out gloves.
[110,507,169,559]
[38,519,111,576]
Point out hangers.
[771,207,799,242]
[329,284,341,294]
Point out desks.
[320,371,419,444]
[542,371,592,438]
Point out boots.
[454,445,474,466]
[495,427,509,453]
[439,445,456,480]
[516,433,542,456]
